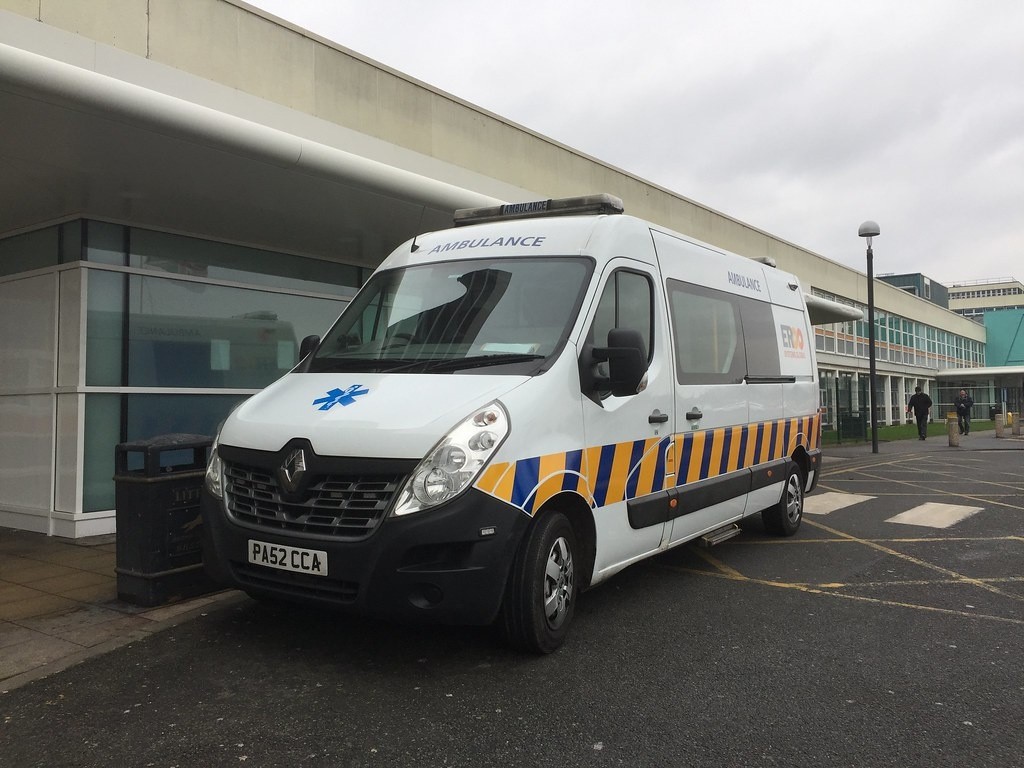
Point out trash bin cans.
[110,431,220,607]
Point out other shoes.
[921,434,926,440]
[964,432,968,435]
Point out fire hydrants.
[1007,412,1012,424]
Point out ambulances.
[202,193,824,656]
[86,310,300,481]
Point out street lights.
[857,219,880,451]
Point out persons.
[954,390,973,435]
[908,387,932,440]
